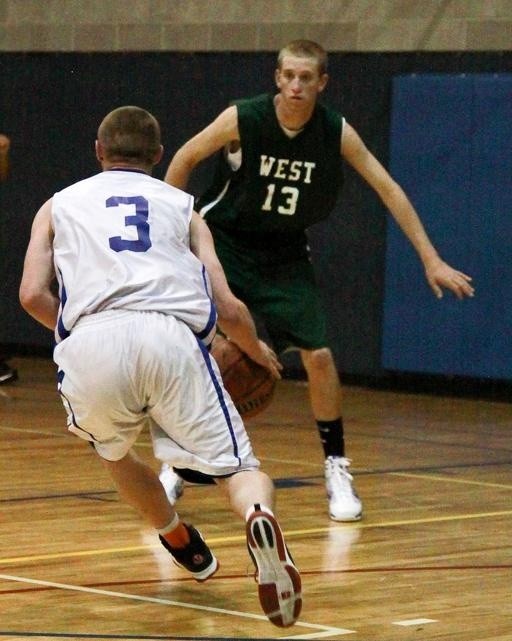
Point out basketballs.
[209,338,275,421]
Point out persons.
[0,134,33,384]
[158,36,476,521]
[18,103,303,628]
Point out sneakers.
[246,503,301,628]
[159,463,184,505]
[159,523,219,581]
[325,455,362,521]
